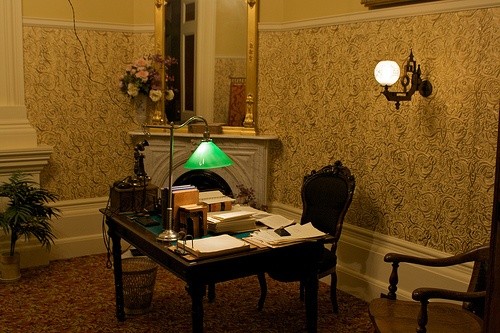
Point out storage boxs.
[188,122,227,134]
[110,183,158,215]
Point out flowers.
[118,53,163,103]
[161,57,180,101]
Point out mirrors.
[154,0,260,136]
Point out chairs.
[256,160,357,314]
[368,244,491,333]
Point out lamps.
[372,48,433,110]
[143,116,234,241]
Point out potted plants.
[0,167,65,281]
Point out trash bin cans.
[119,257,158,314]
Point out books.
[199,190,234,211]
[161,185,207,240]
[206,211,257,234]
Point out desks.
[99,208,335,333]
